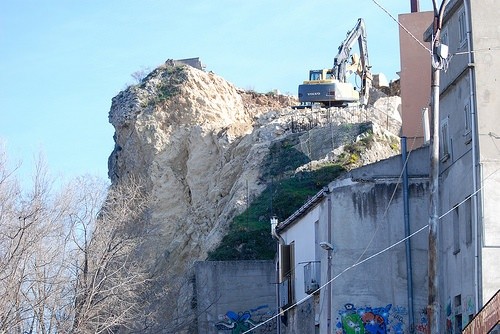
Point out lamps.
[320,242,334,250]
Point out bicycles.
[215,310,282,334]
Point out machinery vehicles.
[291,19,373,106]
